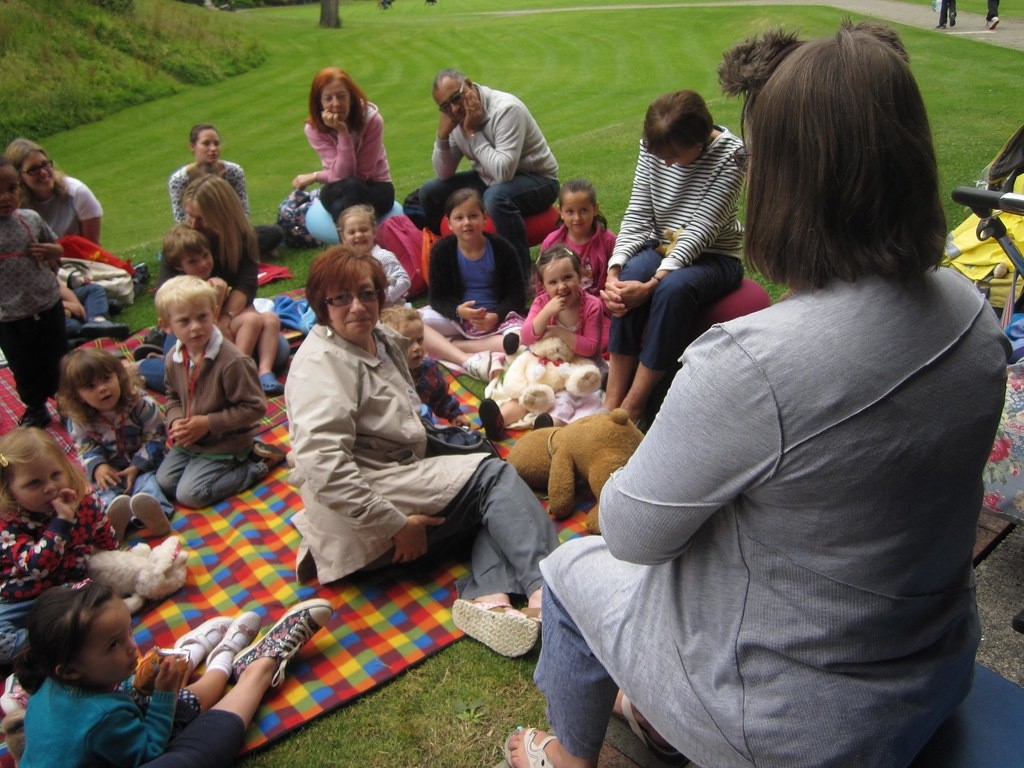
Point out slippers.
[504,726,558,768]
[615,694,681,756]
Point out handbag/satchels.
[375,215,427,300]
[277,185,323,250]
[59,256,134,306]
[420,417,502,460]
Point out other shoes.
[936,24,945,29]
[988,17,1000,30]
[18,405,52,429]
[985,21,991,29]
[81,320,129,338]
[133,344,164,361]
[249,442,286,469]
[106,494,133,543]
[131,263,150,285]
[478,400,504,441]
[0,673,29,715]
[533,412,554,431]
[175,616,234,660]
[130,492,170,537]
[206,611,261,667]
[950,13,956,27]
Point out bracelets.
[222,309,234,319]
[651,274,661,283]
[313,171,317,181]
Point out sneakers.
[234,598,335,686]
[464,350,491,383]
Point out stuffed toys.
[506,413,645,534]
[491,336,602,414]
[649,228,684,258]
[82,534,190,612]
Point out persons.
[419,68,561,288]
[715,20,911,81]
[985,0,1000,30]
[284,247,563,657]
[0,137,130,432]
[1,426,333,768]
[338,177,616,441]
[501,34,1008,767]
[593,88,746,426]
[59,347,171,540]
[155,274,286,509]
[135,172,291,395]
[936,0,958,28]
[168,123,285,259]
[291,66,395,244]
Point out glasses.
[18,159,54,175]
[439,81,464,113]
[731,148,753,176]
[320,290,379,308]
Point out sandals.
[260,373,284,393]
[452,598,540,658]
